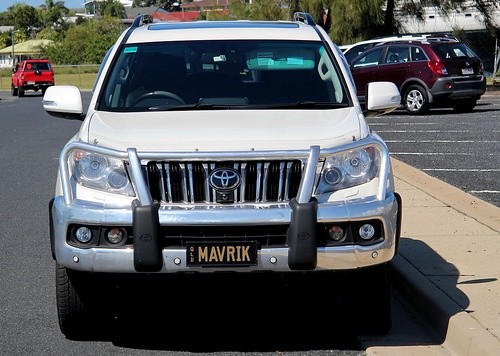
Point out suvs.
[338,34,486,115]
[11,59,54,97]
[42,12,402,335]
